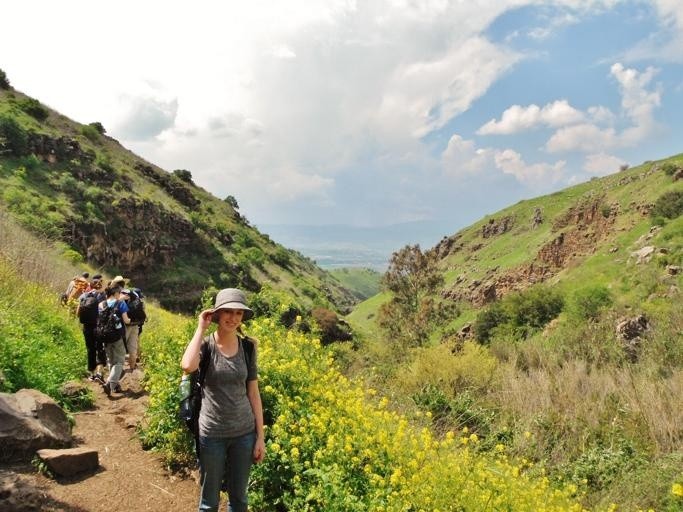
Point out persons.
[180,288,265,512]
[60,271,146,397]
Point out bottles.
[178,374,190,401]
[112,313,122,328]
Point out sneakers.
[90,373,121,396]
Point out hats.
[207,288,254,323]
[110,275,130,288]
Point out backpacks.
[96,301,125,343]
[127,299,146,321]
[79,293,98,314]
[179,338,252,436]
